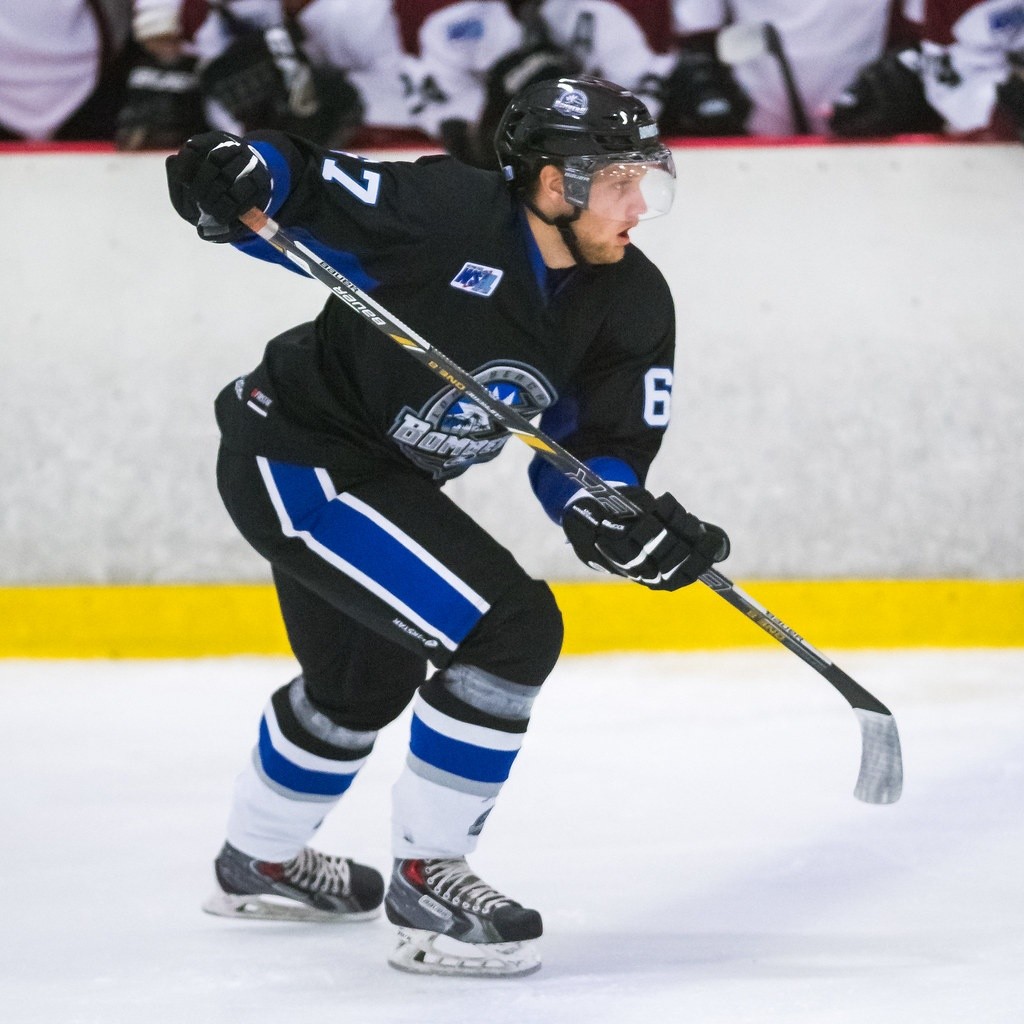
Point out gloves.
[165,128,275,244]
[564,484,730,591]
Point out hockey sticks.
[236,204,905,806]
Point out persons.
[164,73,731,979]
[1,0,1024,144]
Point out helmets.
[494,75,663,165]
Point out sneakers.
[201,840,385,920]
[385,854,543,977]
